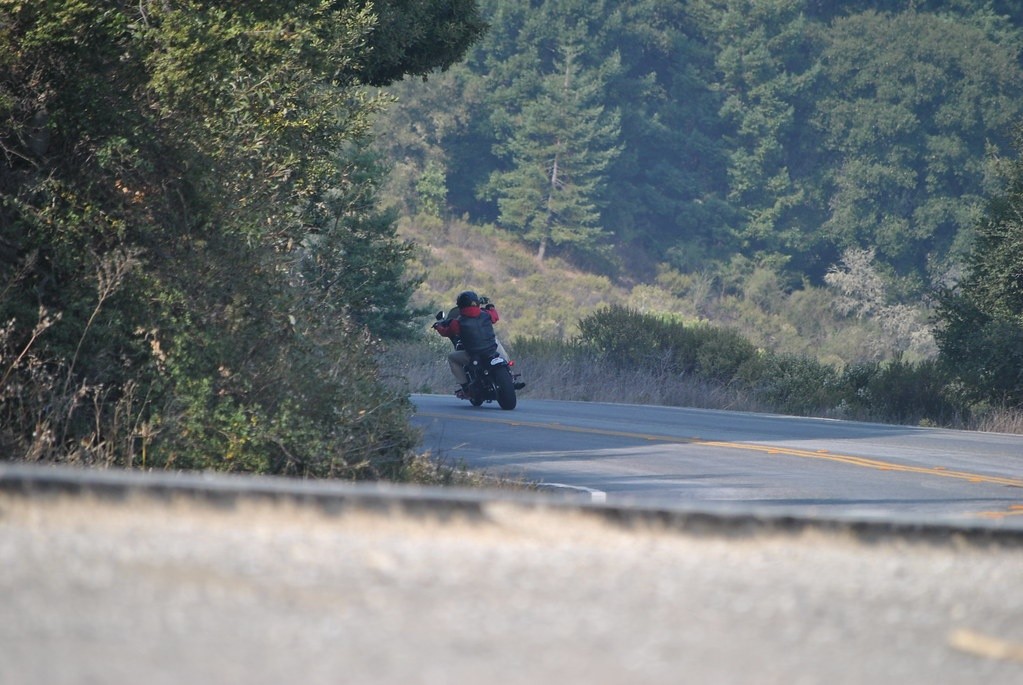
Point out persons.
[433,291,511,400]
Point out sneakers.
[456,390,472,399]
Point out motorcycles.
[431,295,525,411]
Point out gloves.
[433,322,440,329]
[486,304,494,310]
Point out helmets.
[456,291,479,308]
